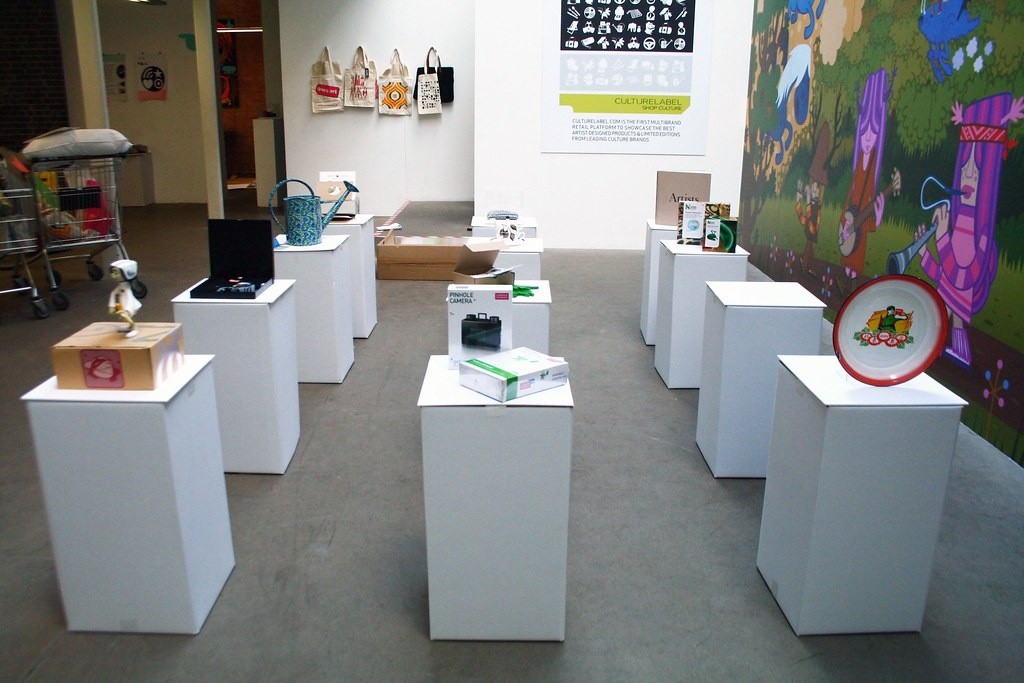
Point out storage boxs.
[452,243,515,285]
[51,321,186,391]
[458,347,569,402]
[189,218,274,300]
[702,216,738,253]
[321,200,361,214]
[676,202,731,245]
[447,283,513,373]
[655,170,712,226]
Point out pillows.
[22,128,133,158]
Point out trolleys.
[0,153,148,319]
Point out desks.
[120,153,154,207]
[271,233,355,384]
[694,281,827,478]
[415,354,574,643]
[639,220,679,346]
[170,278,301,475]
[756,354,968,637]
[513,279,552,354]
[470,216,538,239]
[19,352,236,637]
[325,213,378,338]
[467,238,544,280]
[654,239,751,389]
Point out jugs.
[269,178,359,246]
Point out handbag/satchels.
[417,47,444,113]
[413,47,454,103]
[377,48,413,115]
[311,45,344,112]
[343,45,377,107]
